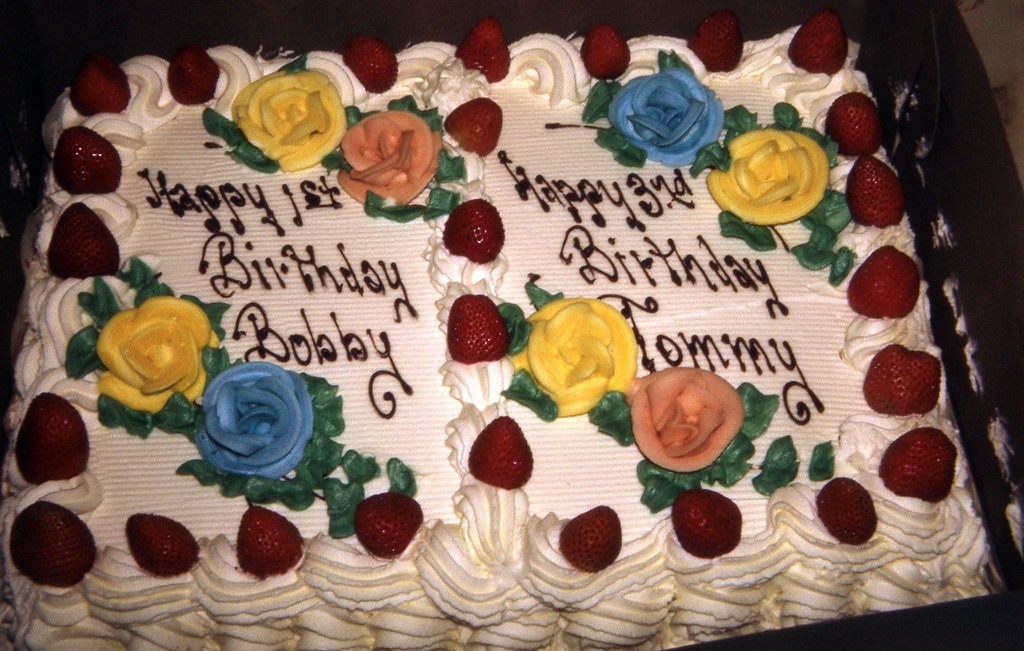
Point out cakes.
[0,6,1024,651]
